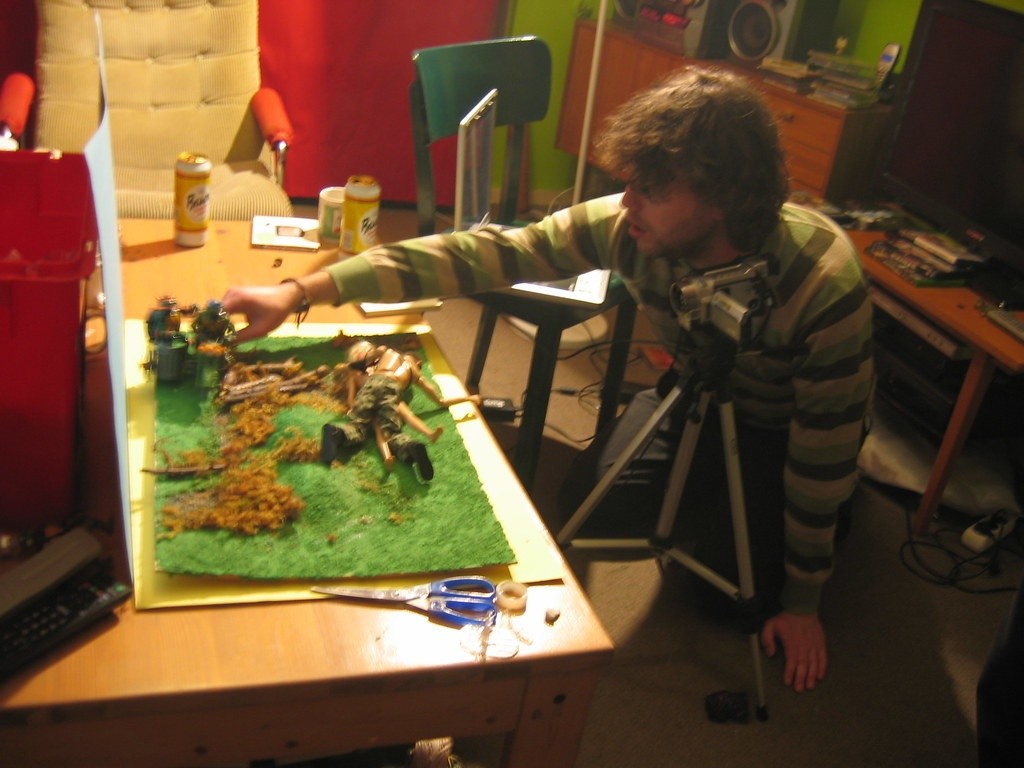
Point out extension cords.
[961,514,1015,555]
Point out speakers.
[726,0,840,74]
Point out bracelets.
[279,279,312,326]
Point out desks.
[0,219,615,768]
[833,202,1024,536]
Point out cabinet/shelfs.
[556,19,895,207]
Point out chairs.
[408,37,637,495]
[0,0,294,219]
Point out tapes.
[497,580,528,611]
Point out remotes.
[987,309,1024,342]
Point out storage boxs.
[0,151,108,526]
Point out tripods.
[556,329,773,724]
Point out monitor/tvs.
[866,0,1024,311]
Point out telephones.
[875,42,903,92]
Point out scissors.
[310,576,497,626]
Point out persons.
[217,64,875,692]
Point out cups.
[318,184,347,245]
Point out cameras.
[671,254,773,347]
